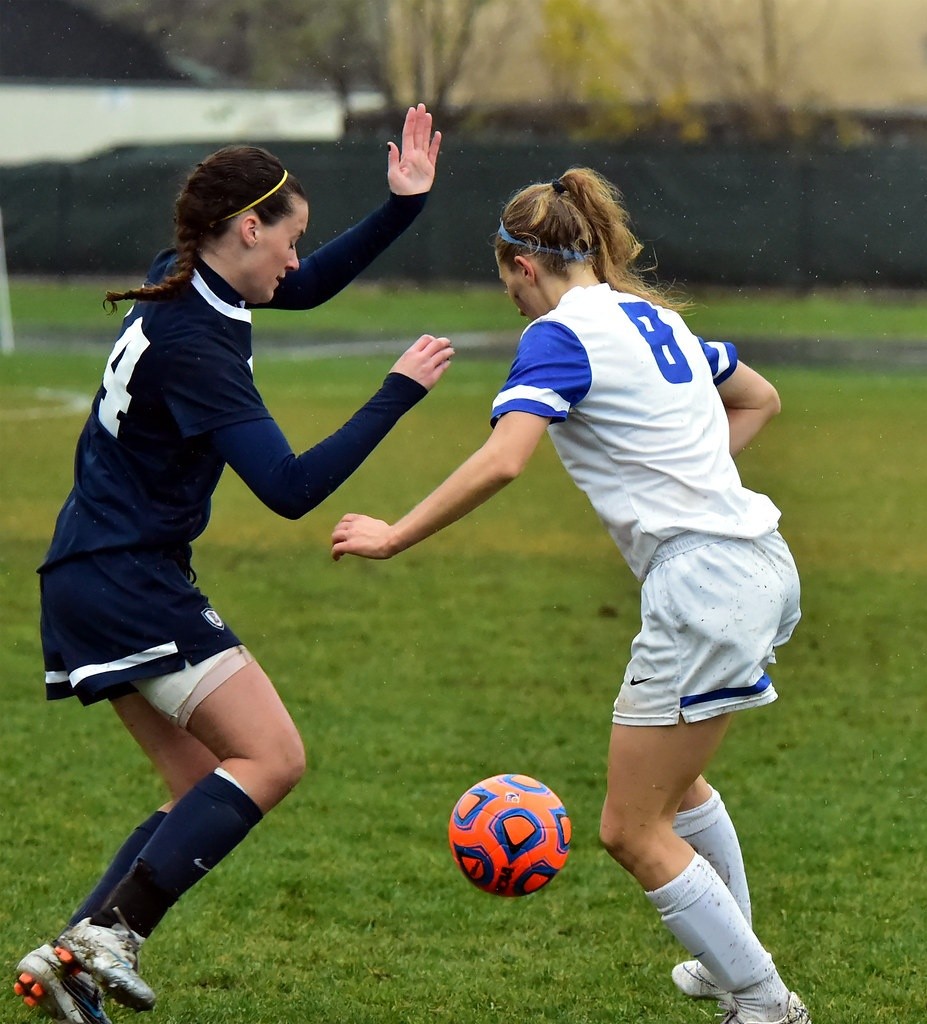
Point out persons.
[331,168,812,1024]
[14,103,456,1024]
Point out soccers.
[448,773,572,897]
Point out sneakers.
[672,961,734,1006]
[54,905,157,1012]
[713,993,813,1024]
[14,941,113,1024]
